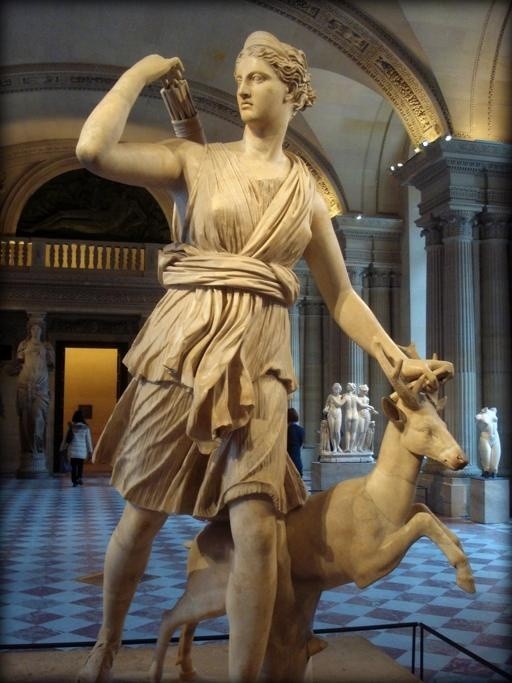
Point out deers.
[147,338,476,677]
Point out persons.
[287,408,305,477]
[323,382,378,452]
[76,28,454,683]
[59,410,92,487]
[476,407,501,477]
[16,318,56,454]
